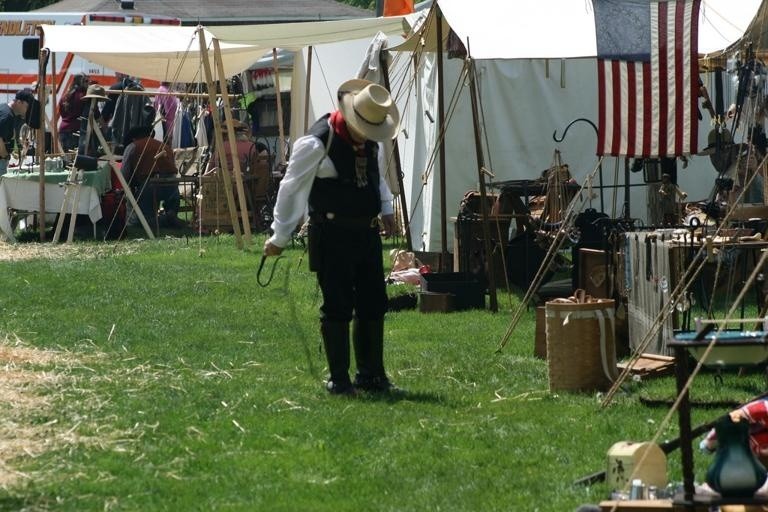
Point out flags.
[590,0,701,158]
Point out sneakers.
[327,378,389,393]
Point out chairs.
[245,134,275,233]
[666,217,768,495]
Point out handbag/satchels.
[26,99,40,129]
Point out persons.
[696,124,749,204]
[262,79,402,397]
[47,72,103,153]
[119,122,180,226]
[214,119,259,174]
[101,70,134,121]
[0,89,35,179]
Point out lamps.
[705,414,767,498]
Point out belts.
[309,213,379,227]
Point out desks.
[0,161,112,245]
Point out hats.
[66,155,97,171]
[696,128,747,156]
[223,117,249,135]
[338,78,400,142]
[16,91,34,104]
[80,84,110,103]
[124,125,155,144]
